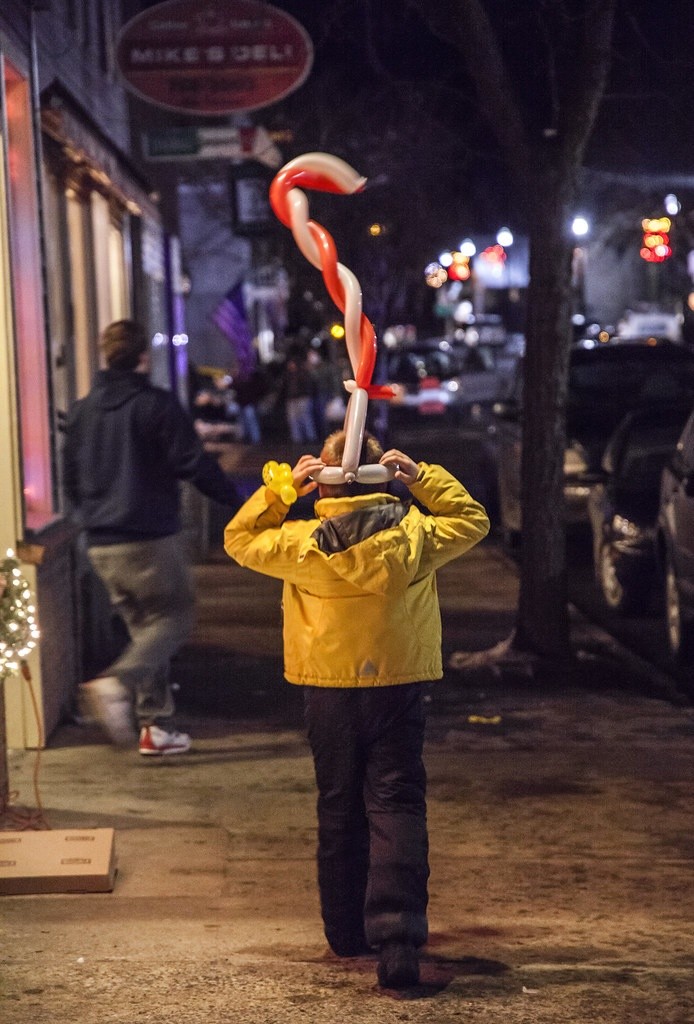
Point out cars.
[291,300,694,681]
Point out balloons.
[268,152,398,485]
[262,460,297,505]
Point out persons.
[277,360,320,443]
[226,360,263,442]
[61,319,247,757]
[223,431,491,998]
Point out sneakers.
[79,677,138,746]
[139,724,192,755]
